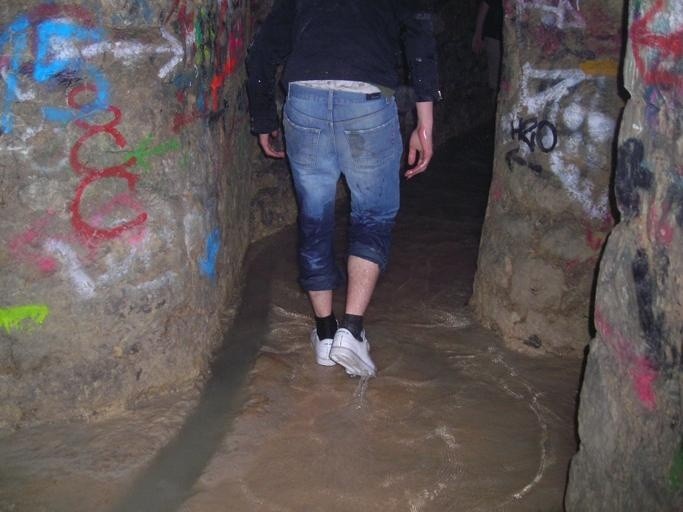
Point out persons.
[247,1,435,379]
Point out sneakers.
[310,328,378,378]
